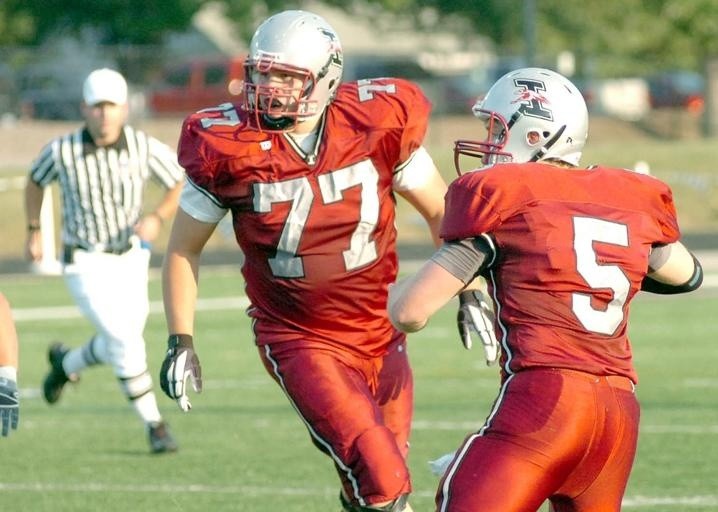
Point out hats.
[83,67,127,107]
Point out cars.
[1,56,250,123]
[341,55,709,128]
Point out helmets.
[240,10,344,135]
[452,67,589,176]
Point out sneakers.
[148,415,177,454]
[44,341,74,404]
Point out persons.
[0,291,22,440]
[158,8,504,512]
[385,66,705,511]
[22,63,190,454]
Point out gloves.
[0,366,19,436]
[457,289,503,366]
[160,333,203,413]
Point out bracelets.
[26,224,41,232]
